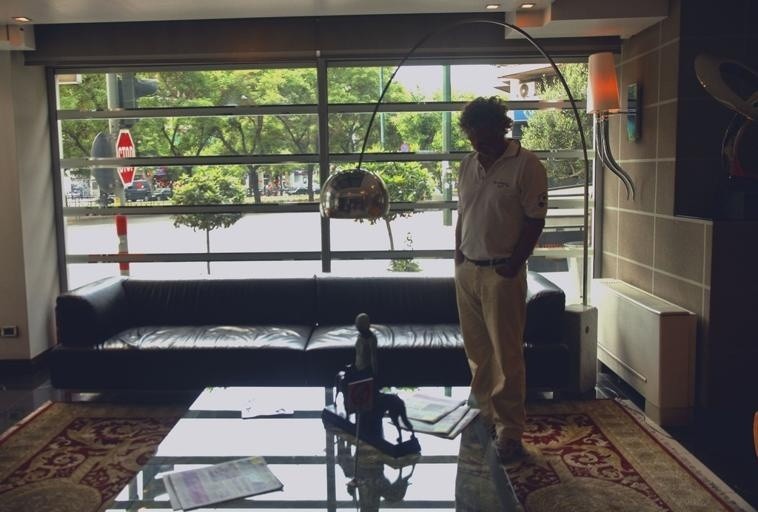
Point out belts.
[463,256,510,267]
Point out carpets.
[1,399,756,512]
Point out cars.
[151,187,173,201]
[290,186,317,195]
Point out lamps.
[320,15,597,405]
[583,48,639,206]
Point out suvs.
[123,180,154,203]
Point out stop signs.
[114,128,138,188]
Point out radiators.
[587,277,698,425]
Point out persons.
[354,313,378,371]
[455,97,547,462]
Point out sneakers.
[495,436,525,473]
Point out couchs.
[46,272,566,407]
